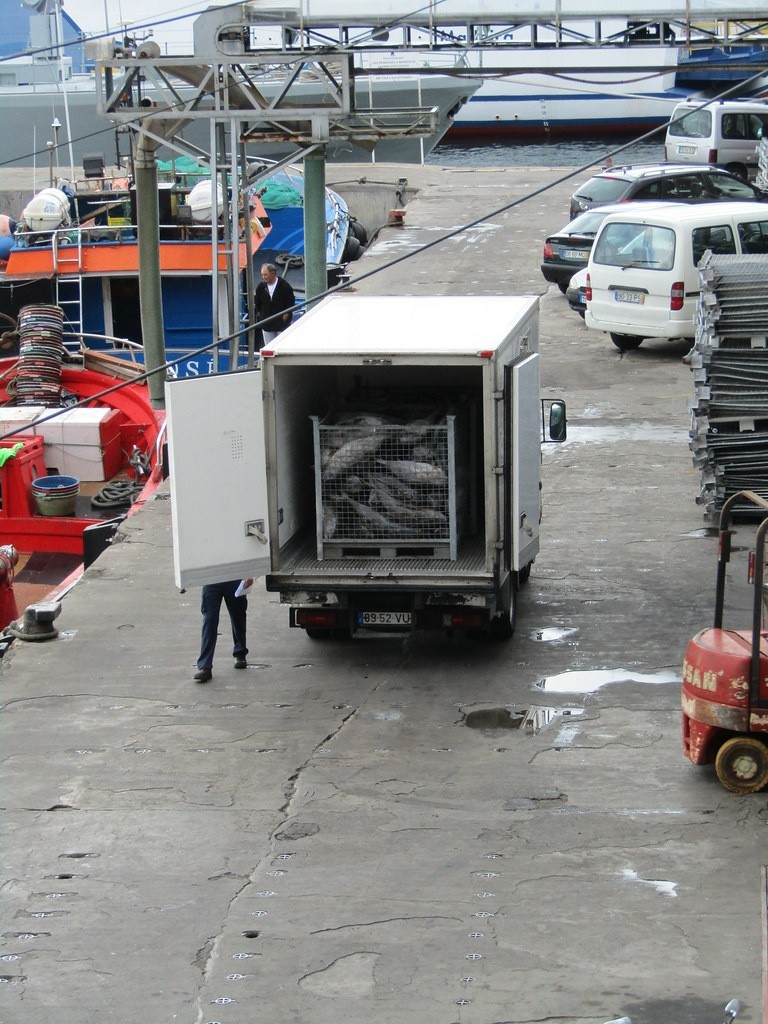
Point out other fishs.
[321,411,448,538]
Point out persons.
[255,263,294,346]
[193,578,252,681]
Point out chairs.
[688,183,703,198]
[667,181,678,198]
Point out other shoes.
[233,656,248,668]
[193,669,212,680]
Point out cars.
[541,201,691,295]
[567,225,753,320]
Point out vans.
[663,96,768,183]
[583,197,768,351]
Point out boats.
[1,1,768,198]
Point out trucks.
[162,295,569,643]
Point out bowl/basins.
[31,475,80,516]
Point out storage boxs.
[0,406,111,482]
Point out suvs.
[570,162,768,222]
